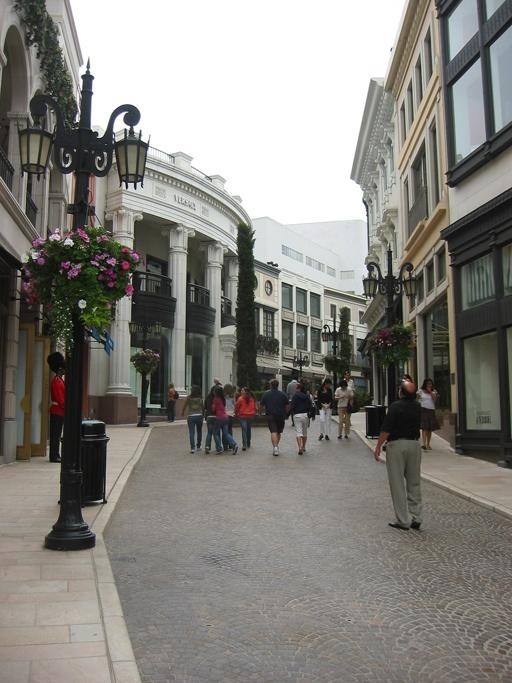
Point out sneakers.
[205,446,209,454]
[216,451,220,454]
[197,448,202,451]
[232,445,239,455]
[273,447,279,456]
[189,450,195,454]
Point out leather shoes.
[50,456,62,462]
[344,435,348,439]
[318,434,323,441]
[388,522,409,531]
[325,435,329,440]
[410,522,421,530]
[338,435,342,439]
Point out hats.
[47,352,65,370]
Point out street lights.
[362,243,418,405]
[322,312,349,416]
[293,352,309,384]
[15,57,151,551]
[128,310,162,427]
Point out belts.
[387,436,419,442]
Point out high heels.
[298,448,303,455]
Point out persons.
[306,389,315,421]
[213,376,223,387]
[48,363,66,463]
[223,384,235,451]
[416,379,438,452]
[335,380,354,439]
[181,384,205,454]
[401,375,413,384]
[205,384,224,453]
[344,371,355,427]
[211,386,238,456]
[374,381,424,531]
[317,378,333,442]
[287,375,301,427]
[166,382,179,423]
[258,379,289,456]
[235,386,258,451]
[291,384,315,456]
[313,390,318,415]
